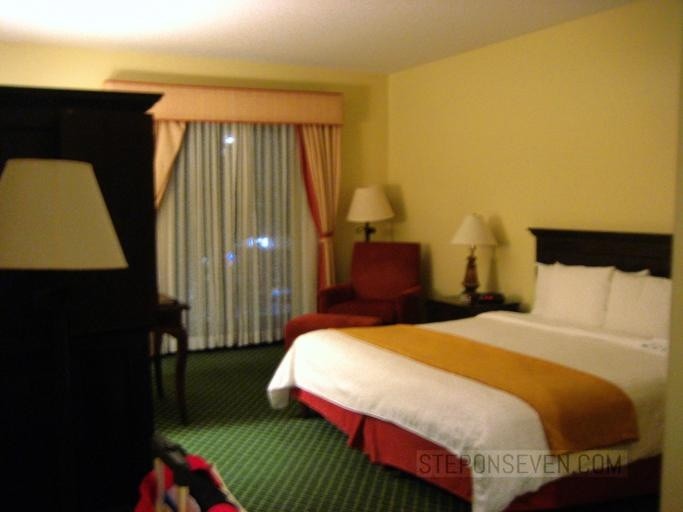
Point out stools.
[285,312,384,352]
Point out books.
[464,293,503,303]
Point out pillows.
[528,259,672,342]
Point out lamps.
[449,214,500,302]
[0,160,128,512]
[345,184,394,243]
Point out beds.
[265,229,674,512]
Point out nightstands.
[424,295,523,324]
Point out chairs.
[314,241,426,325]
